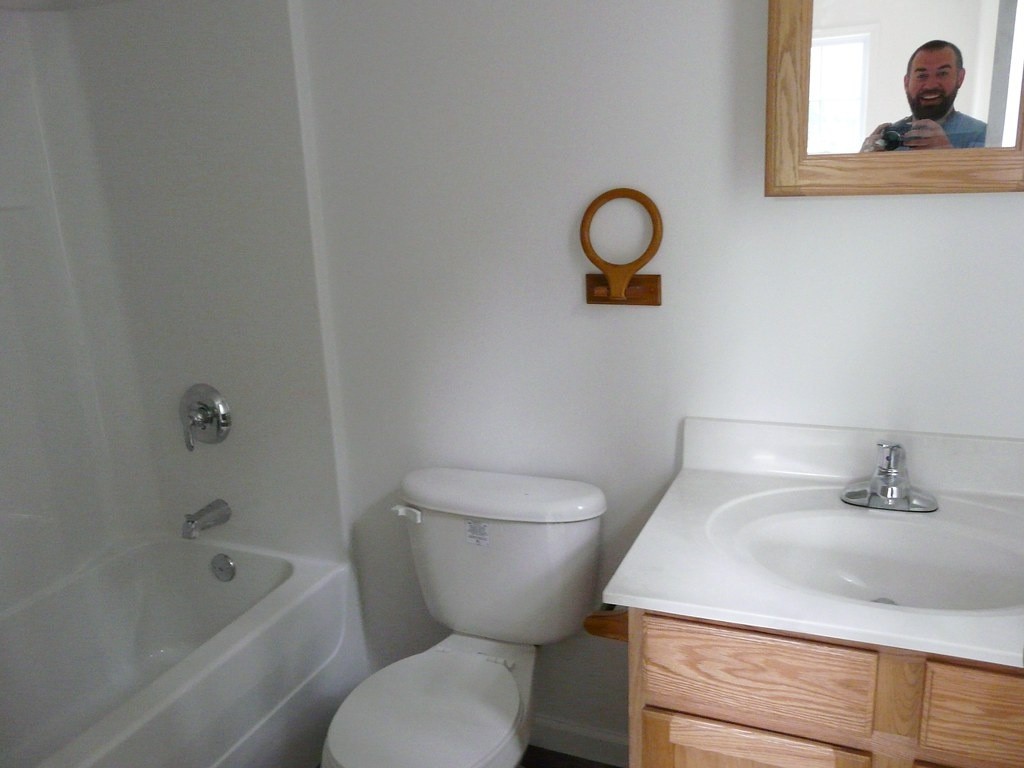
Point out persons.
[859,40,988,153]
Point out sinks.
[702,458,1023,617]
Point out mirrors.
[764,0,1024,198]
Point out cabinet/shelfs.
[626,606,1024,768]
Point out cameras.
[883,126,915,151]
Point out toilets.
[320,465,608,768]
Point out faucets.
[181,497,233,541]
[864,441,914,506]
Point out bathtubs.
[0,534,373,768]
